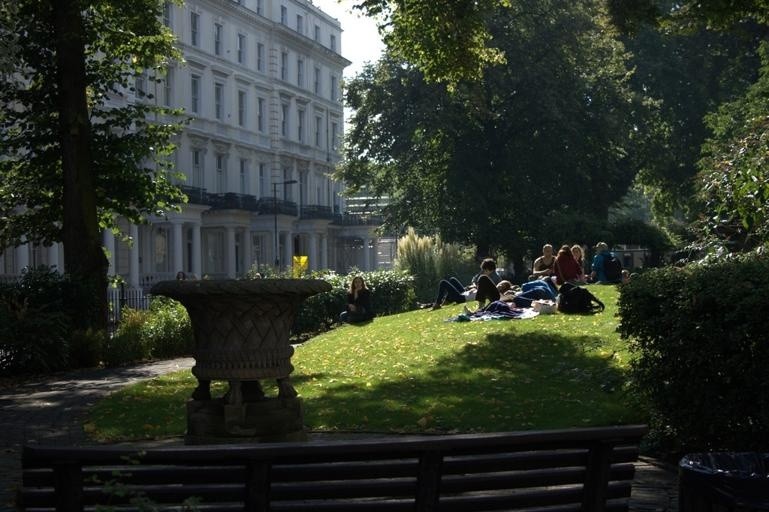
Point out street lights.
[270,179,299,273]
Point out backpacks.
[557,282,605,314]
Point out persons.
[340,276,373,323]
[176,271,186,281]
[414,241,633,316]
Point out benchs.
[17,424,649,512]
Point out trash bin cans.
[678,451,769,512]
